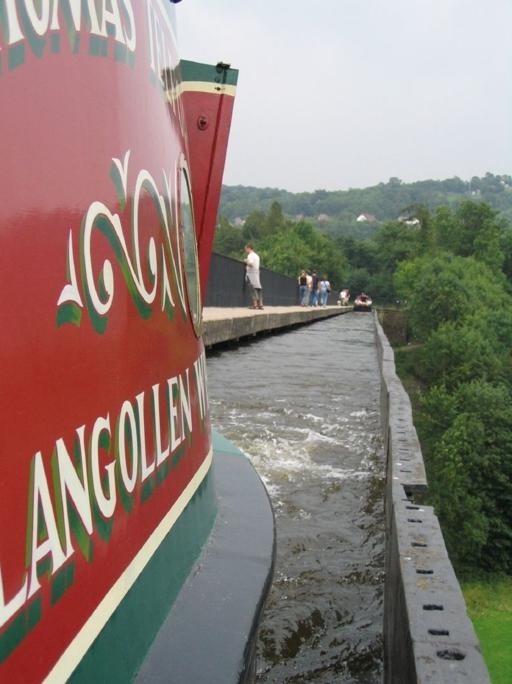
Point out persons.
[242,242,265,310]
[297,268,372,307]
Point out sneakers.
[248,305,264,310]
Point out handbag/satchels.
[326,287,332,293]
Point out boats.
[353,299,373,312]
[0,0,279,684]
